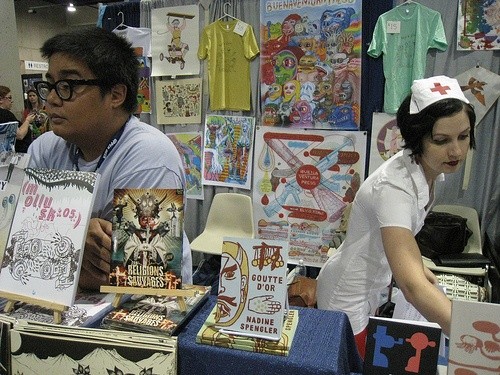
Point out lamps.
[67,2,77,11]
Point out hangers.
[379,0,441,19]
[204,2,252,31]
[112,12,144,35]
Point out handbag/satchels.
[414,210,473,271]
[288,275,317,308]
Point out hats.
[27,88,37,94]
[410,75,470,114]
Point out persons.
[0,86,37,152]
[26,27,193,289]
[22,89,49,148]
[317,76,477,361]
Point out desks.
[0,293,360,375]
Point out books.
[363,315,443,375]
[101,279,211,336]
[9,277,134,328]
[196,300,299,357]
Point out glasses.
[33,78,117,102]
[0,95,12,100]
[29,95,35,97]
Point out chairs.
[190,193,254,279]
[423,204,493,304]
